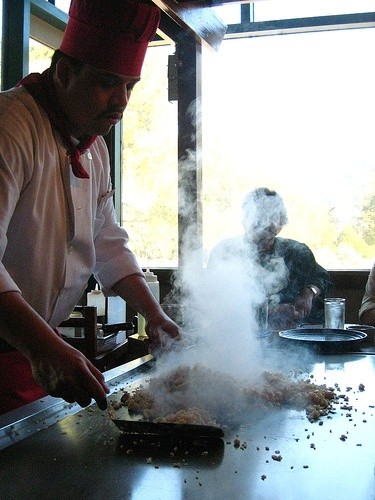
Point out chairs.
[58,305,134,370]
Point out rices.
[61,360,375,484]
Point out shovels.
[97,390,228,445]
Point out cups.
[323,297,346,331]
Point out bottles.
[107,296,126,344]
[87,283,105,337]
[137,266,160,336]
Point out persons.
[0,0,184,411]
[359,262,375,327]
[207,188,332,325]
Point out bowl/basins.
[279,328,368,355]
[346,324,375,343]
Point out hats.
[59,0,160,79]
